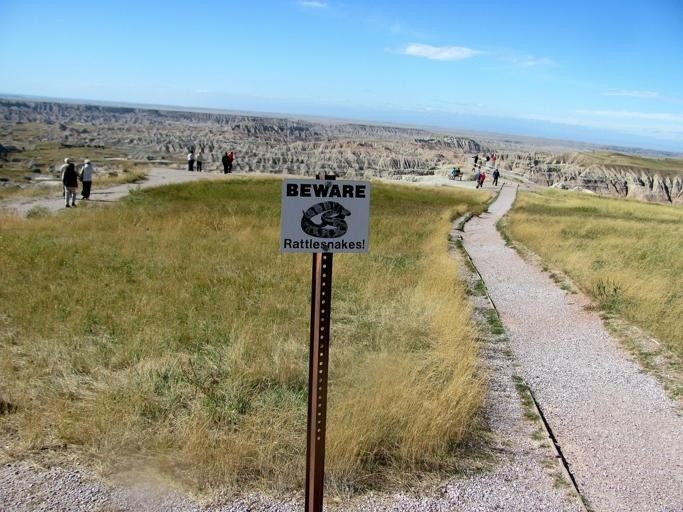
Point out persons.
[187,150,205,172]
[451,167,461,177]
[471,153,501,188]
[60,157,94,208]
[221,151,235,174]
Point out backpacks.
[63,167,76,187]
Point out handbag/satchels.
[79,168,83,181]
